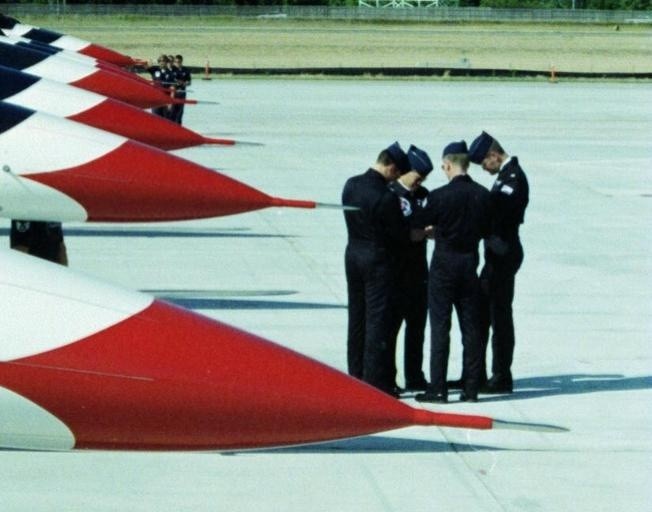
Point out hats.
[388,141,412,175]
[408,144,433,177]
[442,140,469,156]
[469,131,494,164]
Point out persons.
[404,139,493,403]
[388,144,436,393]
[142,52,191,125]
[448,131,529,393]
[342,141,410,400]
[11,220,68,264]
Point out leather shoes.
[391,378,514,403]
